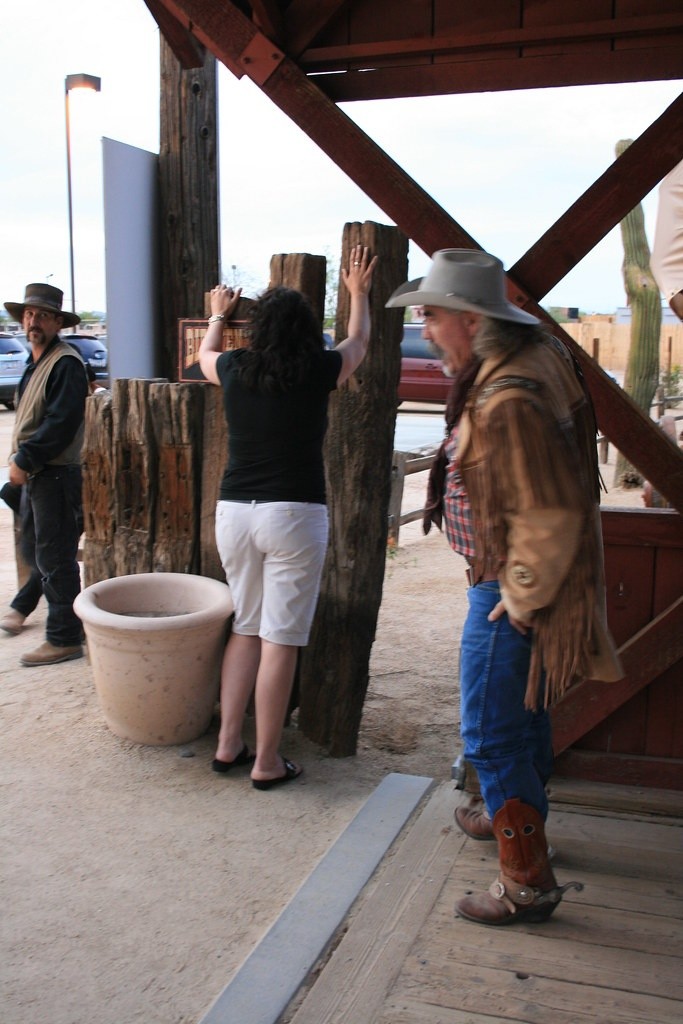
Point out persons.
[0,282,107,665]
[196,246,380,788]
[385,247,626,926]
[652,163,683,319]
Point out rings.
[353,261,360,267]
[214,289,219,292]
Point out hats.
[384,248,542,324]
[3,283,81,328]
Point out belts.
[465,567,499,588]
[226,500,269,503]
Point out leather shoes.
[0,607,25,634]
[18,640,83,666]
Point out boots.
[454,806,498,840]
[454,799,584,926]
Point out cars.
[397,321,458,407]
[57,334,109,382]
[0,330,31,410]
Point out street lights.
[64,73,104,335]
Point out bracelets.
[208,315,224,324]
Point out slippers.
[252,756,303,791]
[211,743,257,772]
